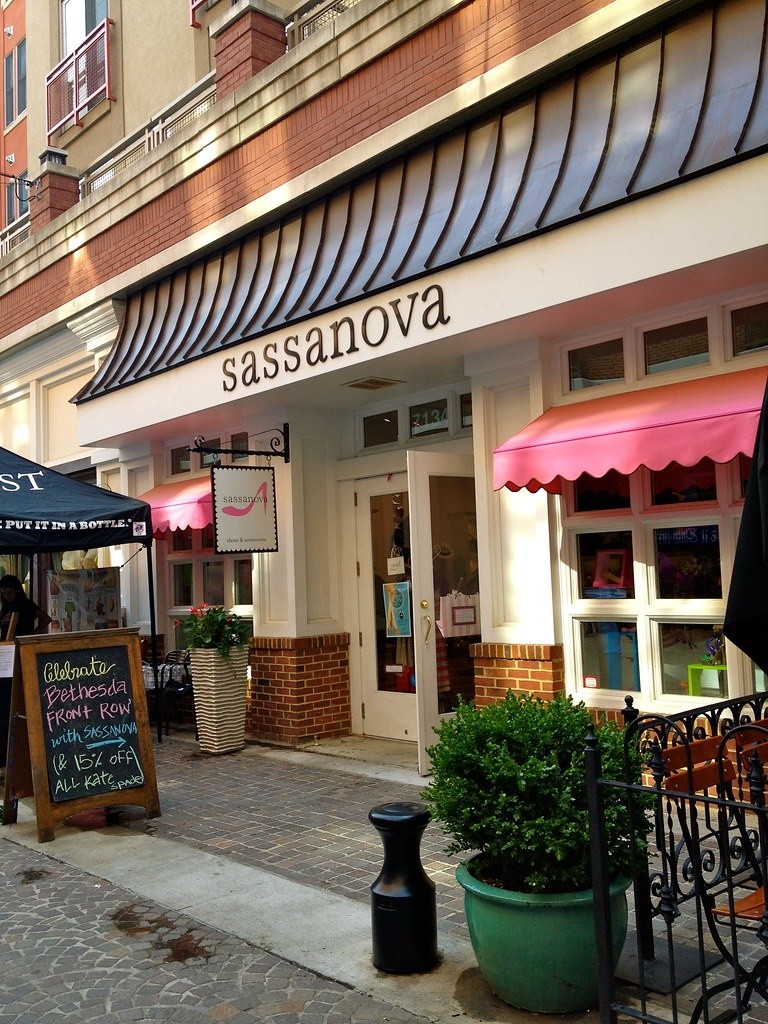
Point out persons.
[1,574,53,767]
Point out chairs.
[664,737,768,1024]
[734,717,768,812]
[141,650,199,743]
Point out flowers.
[170,602,253,673]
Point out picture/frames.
[452,606,477,625]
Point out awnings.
[132,472,216,539]
[489,363,767,496]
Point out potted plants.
[422,690,655,1013]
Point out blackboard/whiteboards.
[18,627,164,825]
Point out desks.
[143,666,191,689]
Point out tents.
[0,445,165,745]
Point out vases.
[186,647,249,755]
